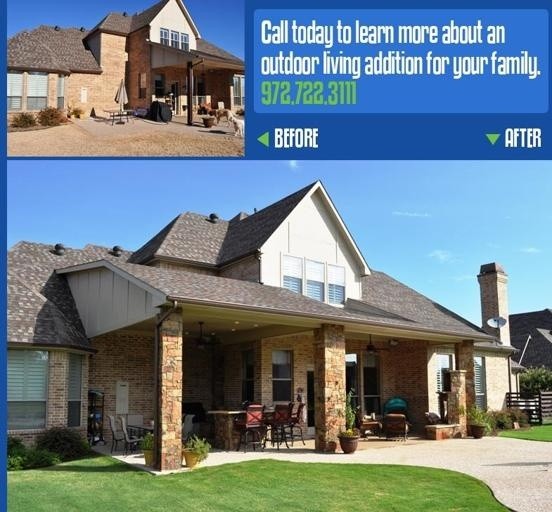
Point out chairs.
[355,405,382,439]
[106,415,144,456]
[182,402,210,444]
[234,402,305,453]
[384,413,412,441]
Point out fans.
[183,321,221,351]
[352,334,390,355]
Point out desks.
[127,425,155,437]
[207,409,275,450]
[103,110,134,126]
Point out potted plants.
[465,403,488,438]
[182,433,212,468]
[335,387,361,454]
[139,431,155,466]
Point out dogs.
[229,116,245,138]
[209,110,239,128]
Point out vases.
[202,118,215,128]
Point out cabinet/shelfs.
[87,389,107,447]
